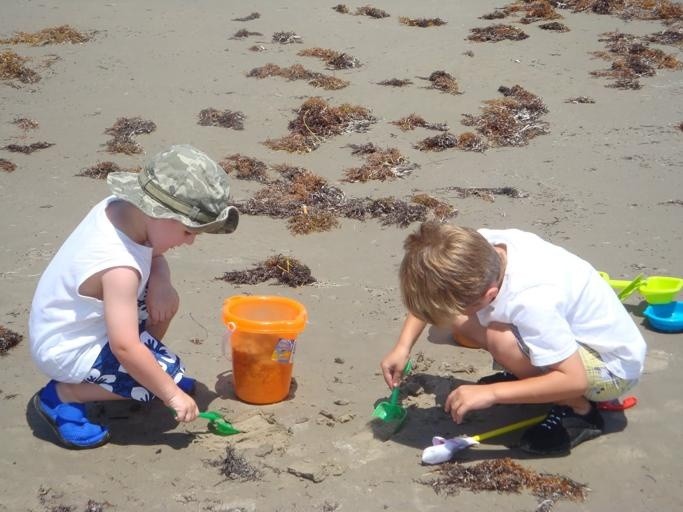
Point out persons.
[382,219,647,456]
[29,143,239,450]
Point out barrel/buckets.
[222,294,308,405]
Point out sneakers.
[521,397,605,456]
[31,378,111,451]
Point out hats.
[105,142,240,236]
